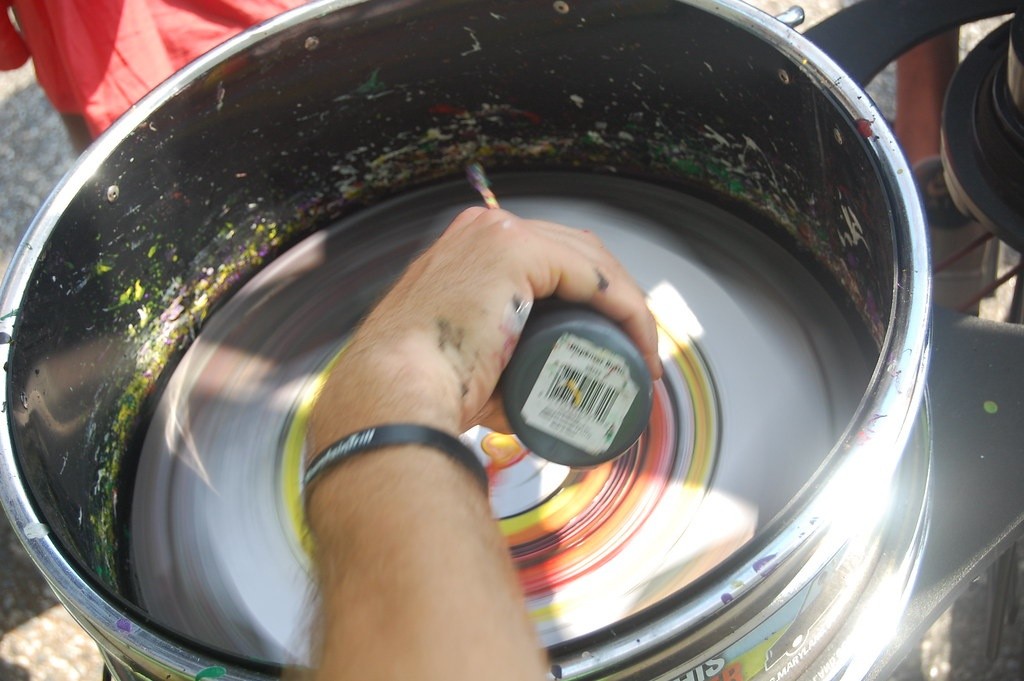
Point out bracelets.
[304,423,489,490]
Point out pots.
[0,0,937,681]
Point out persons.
[302,205,662,681]
[887,26,978,232]
